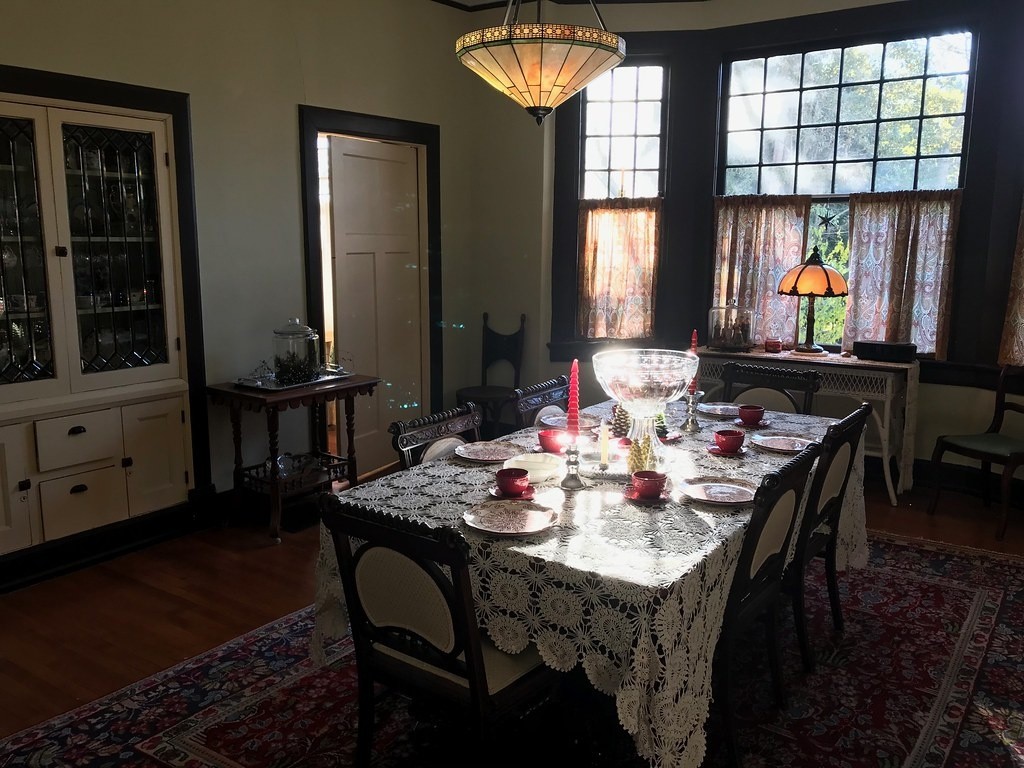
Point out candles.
[566,359,579,441]
[686,329,698,394]
[600,423,608,464]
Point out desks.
[207,369,381,548]
[318,396,872,768]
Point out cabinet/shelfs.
[0,97,213,591]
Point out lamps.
[778,245,848,353]
[456,0,625,125]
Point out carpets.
[2,533,1024,768]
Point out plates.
[454,401,813,536]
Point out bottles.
[765,337,782,352]
[272,317,320,385]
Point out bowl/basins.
[738,405,764,425]
[632,470,667,500]
[538,430,565,452]
[496,468,530,496]
[0,290,142,309]
[715,430,744,452]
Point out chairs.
[927,363,1024,541]
[510,376,570,430]
[320,494,569,768]
[387,402,481,469]
[454,310,526,441]
[707,400,871,730]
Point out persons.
[721,317,744,345]
[739,316,750,344]
[714,320,720,341]
